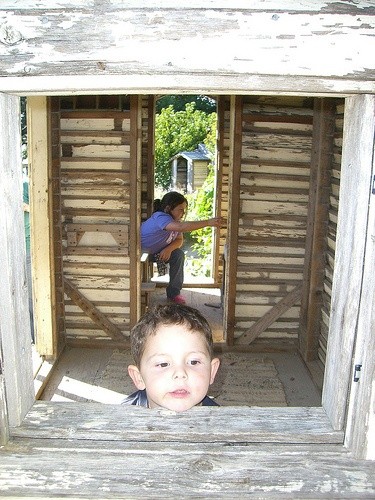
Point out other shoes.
[167,293,186,305]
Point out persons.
[142,192,226,304]
[120,304,220,411]
